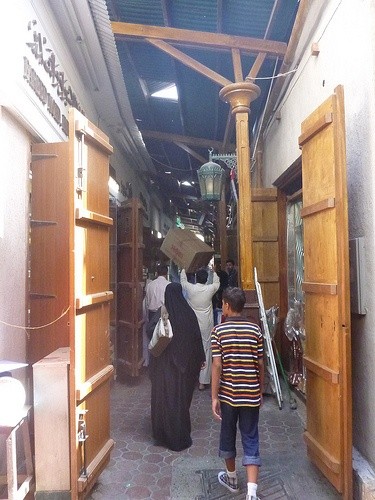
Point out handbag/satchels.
[147,303,174,359]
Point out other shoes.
[199,384,205,391]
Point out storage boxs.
[161,229,215,273]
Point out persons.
[147,264,170,340]
[180,265,220,391]
[169,259,238,326]
[146,282,206,452]
[142,265,153,370]
[210,287,264,500]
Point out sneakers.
[245,494,263,500]
[217,470,239,493]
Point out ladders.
[253,266,285,410]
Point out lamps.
[196,146,256,201]
